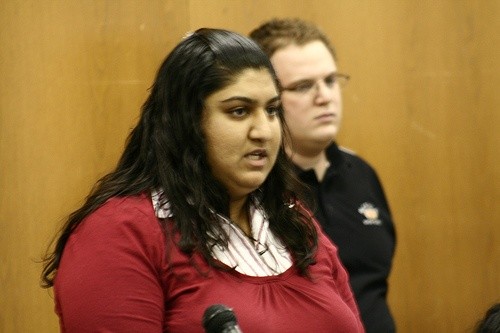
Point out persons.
[248,18,397,333]
[40,27,366,333]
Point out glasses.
[280,73,349,95]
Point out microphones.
[202,304,242,333]
